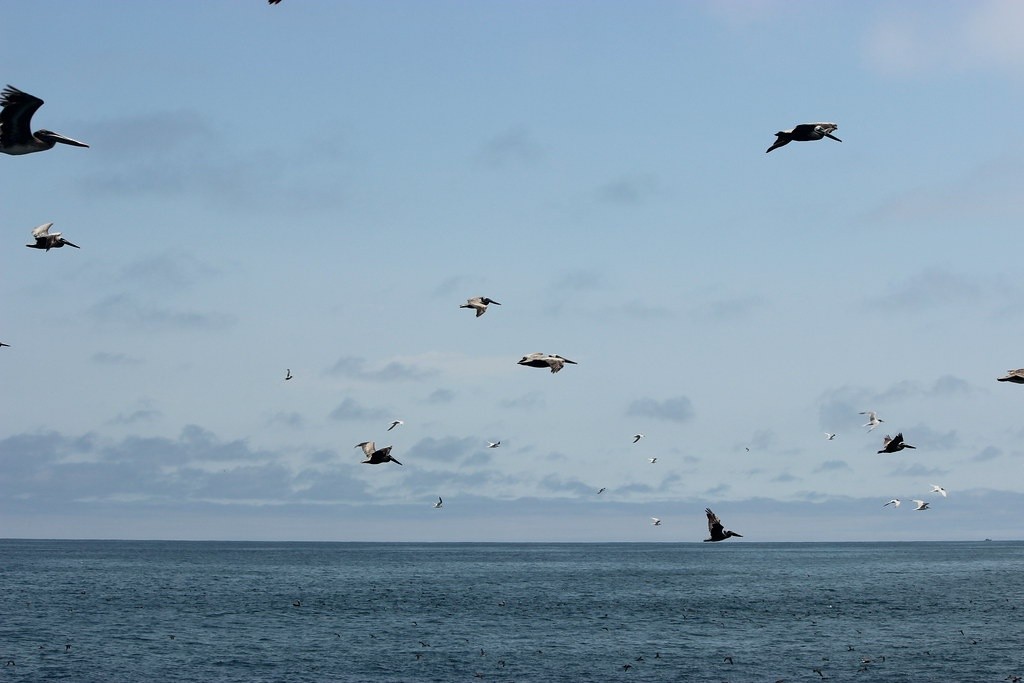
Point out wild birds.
[485,441,501,449]
[632,433,645,443]
[0,84,89,155]
[703,507,743,542]
[354,441,402,465]
[597,488,605,494]
[388,420,403,431]
[648,456,657,463]
[825,432,835,440]
[0,342,10,347]
[746,448,749,452]
[459,296,501,317]
[651,518,661,525]
[286,369,292,381]
[859,411,884,432]
[517,352,578,374]
[878,433,916,454]
[883,484,946,510]
[997,368,1024,384]
[766,122,842,153]
[433,497,443,508]
[26,223,81,252]
